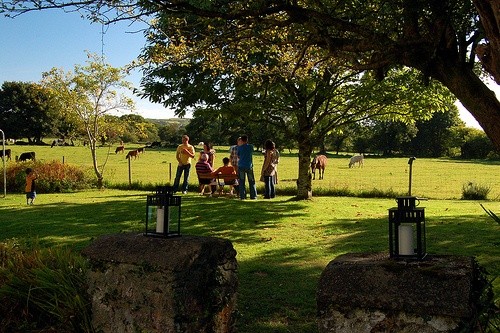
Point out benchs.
[196,171,238,197]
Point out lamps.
[388,196,428,262]
[144,187,181,237]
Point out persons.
[211,157,239,190]
[229,137,241,194]
[199,143,215,192]
[173,135,195,194]
[25,168,39,205]
[237,136,257,199]
[196,154,218,195]
[260,140,278,199]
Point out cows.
[19,151,35,163]
[115,146,125,154]
[0,149,12,162]
[126,147,144,160]
[348,155,363,168]
[311,155,327,181]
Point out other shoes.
[182,190,186,193]
